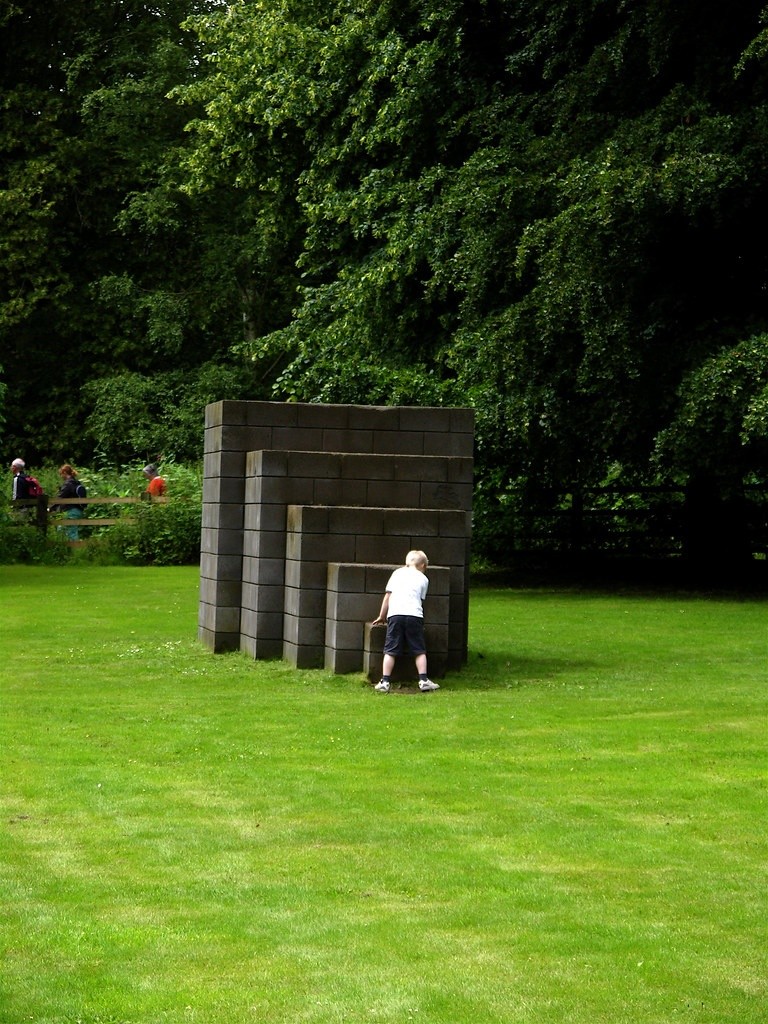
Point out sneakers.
[374,678,390,692]
[418,678,441,692]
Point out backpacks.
[68,480,88,507]
[15,474,44,496]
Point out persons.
[371,551,441,692]
[48,464,82,542]
[143,463,167,497]
[9,458,35,526]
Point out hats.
[142,464,157,475]
